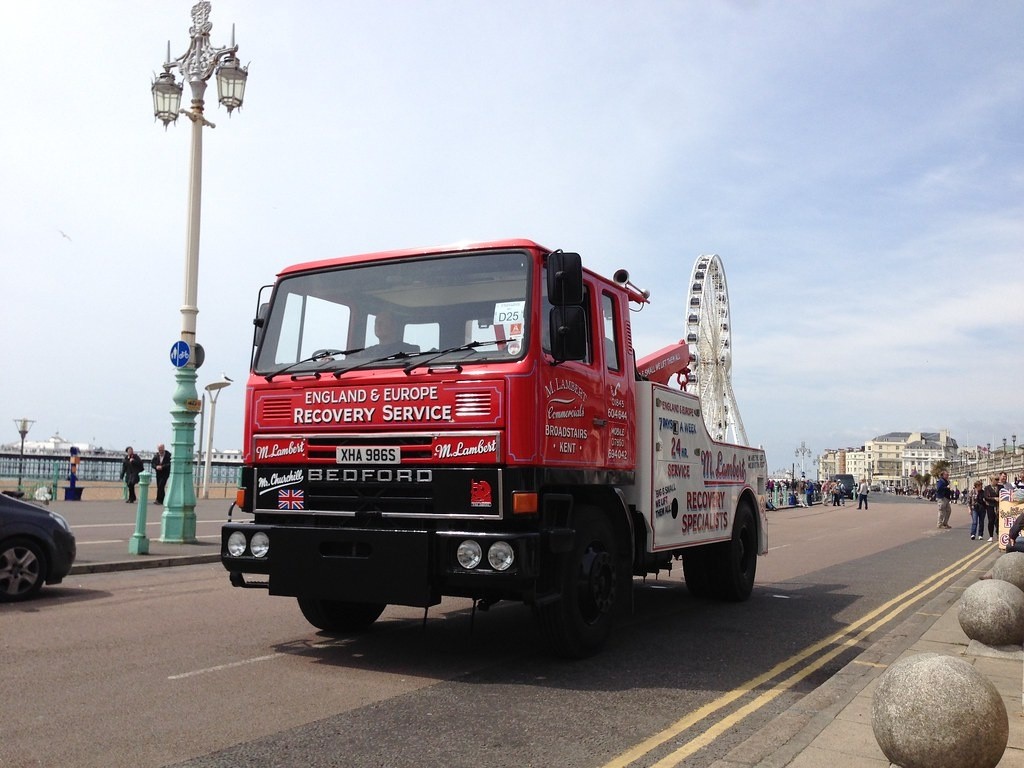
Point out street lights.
[812,457,834,482]
[202,382,231,499]
[16,414,36,491]
[152,1,249,543]
[977,446,981,471]
[794,441,811,478]
[958,451,968,475]
[987,441,990,470]
[1012,433,1016,453]
[1002,437,1007,452]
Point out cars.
[0,492,75,603]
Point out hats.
[801,478,805,481]
[837,479,841,482]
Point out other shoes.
[979,536,984,539]
[937,522,948,529]
[970,534,975,540]
[988,537,992,541]
[945,524,952,529]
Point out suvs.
[834,474,857,500]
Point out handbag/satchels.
[867,488,869,494]
[831,485,836,494]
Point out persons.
[936,471,954,529]
[950,489,967,499]
[829,480,846,506]
[967,470,1024,553]
[151,444,171,505]
[894,485,912,495]
[800,478,821,506]
[346,310,414,358]
[767,479,800,506]
[857,479,868,510]
[925,487,936,502]
[120,447,144,503]
[821,480,830,506]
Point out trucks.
[220,238,768,667]
